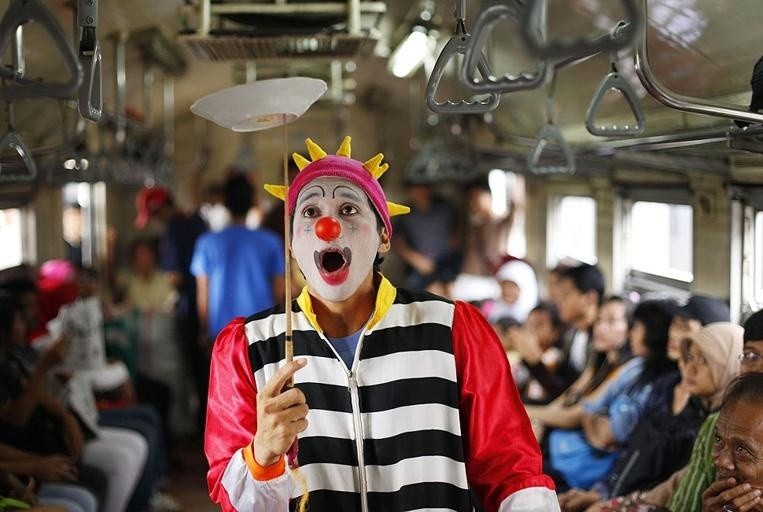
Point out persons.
[0,151,314,512]
[203,133,562,512]
[392,177,762,512]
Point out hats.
[134,185,167,230]
[264,136,411,239]
[670,296,731,326]
[34,259,77,294]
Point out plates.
[190,77,328,133]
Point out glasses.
[739,351,762,361]
